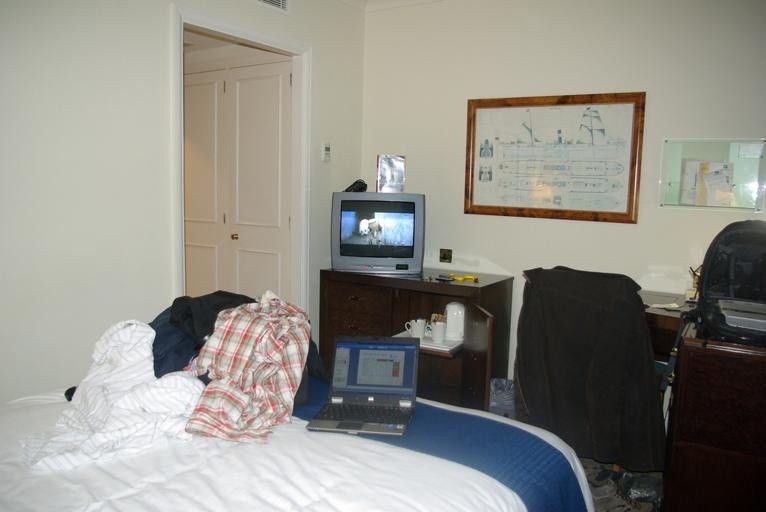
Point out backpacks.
[699,219,765,348]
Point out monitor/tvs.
[330,191,427,277]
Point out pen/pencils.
[690,266,696,275]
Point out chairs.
[517,266,662,479]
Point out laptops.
[304,333,421,437]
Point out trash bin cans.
[488,378,516,421]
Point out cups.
[404,320,426,340]
[431,322,445,343]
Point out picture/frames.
[464,90,647,225]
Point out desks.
[317,266,515,414]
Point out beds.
[0,364,589,512]
